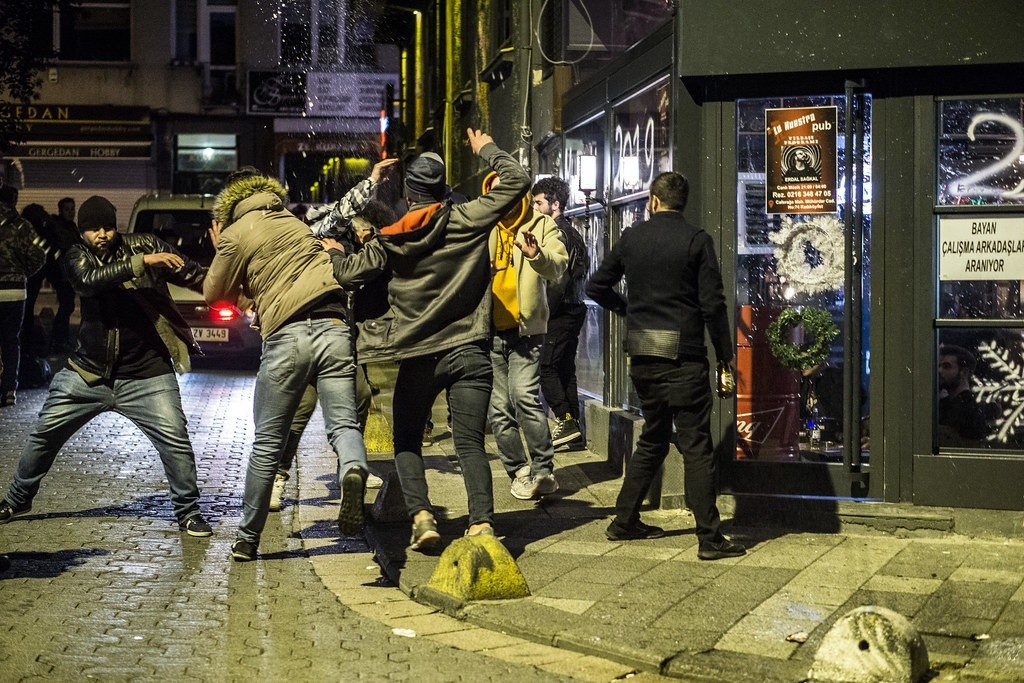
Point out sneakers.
[337,465,365,537]
[551,418,581,448]
[531,471,560,493]
[698,535,745,559]
[0,495,32,523]
[180,514,213,536]
[511,474,542,500]
[605,517,665,540]
[567,436,584,446]
[365,472,384,489]
[231,537,258,560]
[268,473,286,509]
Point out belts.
[267,311,348,337]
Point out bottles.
[716,360,736,399]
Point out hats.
[404,151,446,197]
[77,195,117,233]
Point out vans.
[126,194,261,366]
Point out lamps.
[578,152,610,244]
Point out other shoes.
[446,420,452,432]
[422,421,433,446]
[4,390,18,405]
[409,517,441,551]
[464,525,495,539]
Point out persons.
[0,195,251,536]
[269,128,590,555]
[584,171,748,560]
[203,165,370,561]
[22,197,84,343]
[0,185,46,405]
